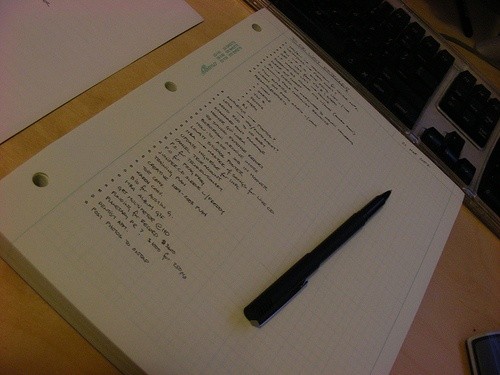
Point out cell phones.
[465,332,500,375]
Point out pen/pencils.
[243,188,395,327]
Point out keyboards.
[244,0,500,238]
